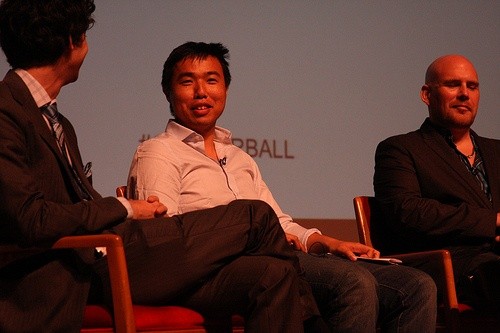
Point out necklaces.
[466,134,477,158]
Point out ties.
[42,102,94,200]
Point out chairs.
[53,186,469,333]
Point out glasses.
[308,242,329,256]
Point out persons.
[0,0,319,333]
[127,40,438,333]
[373,54,500,333]
